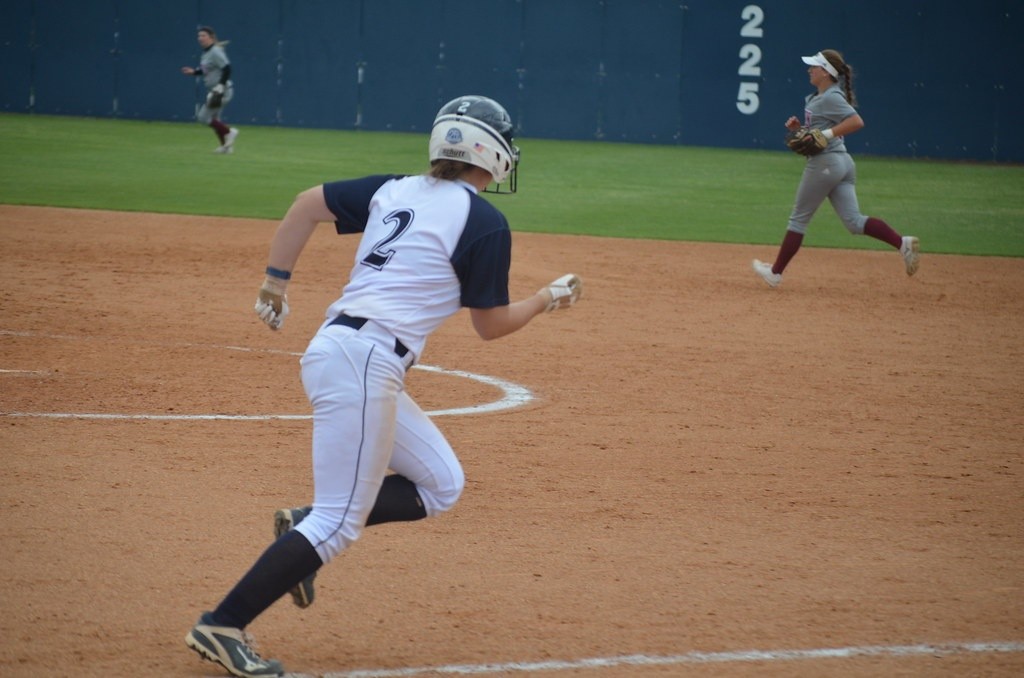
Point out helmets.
[428,95,518,196]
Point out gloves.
[542,273,585,314]
[253,267,291,330]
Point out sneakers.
[184,610,284,678]
[902,235,920,276]
[273,507,318,609]
[214,128,239,153]
[753,259,782,287]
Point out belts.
[327,314,415,371]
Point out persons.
[750,49,920,289]
[182,25,238,154]
[184,95,583,678]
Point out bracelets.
[265,266,291,280]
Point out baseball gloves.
[788,127,830,155]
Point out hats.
[801,50,839,78]
